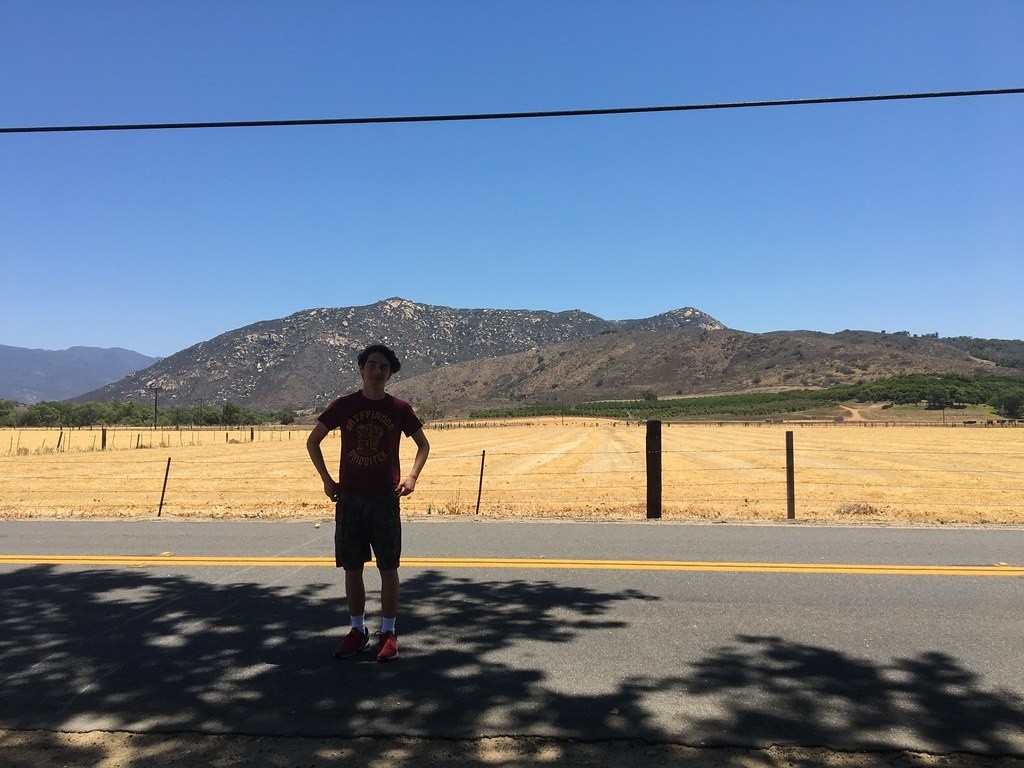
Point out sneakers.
[333,626,369,657]
[374,631,398,660]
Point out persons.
[306,345,430,661]
